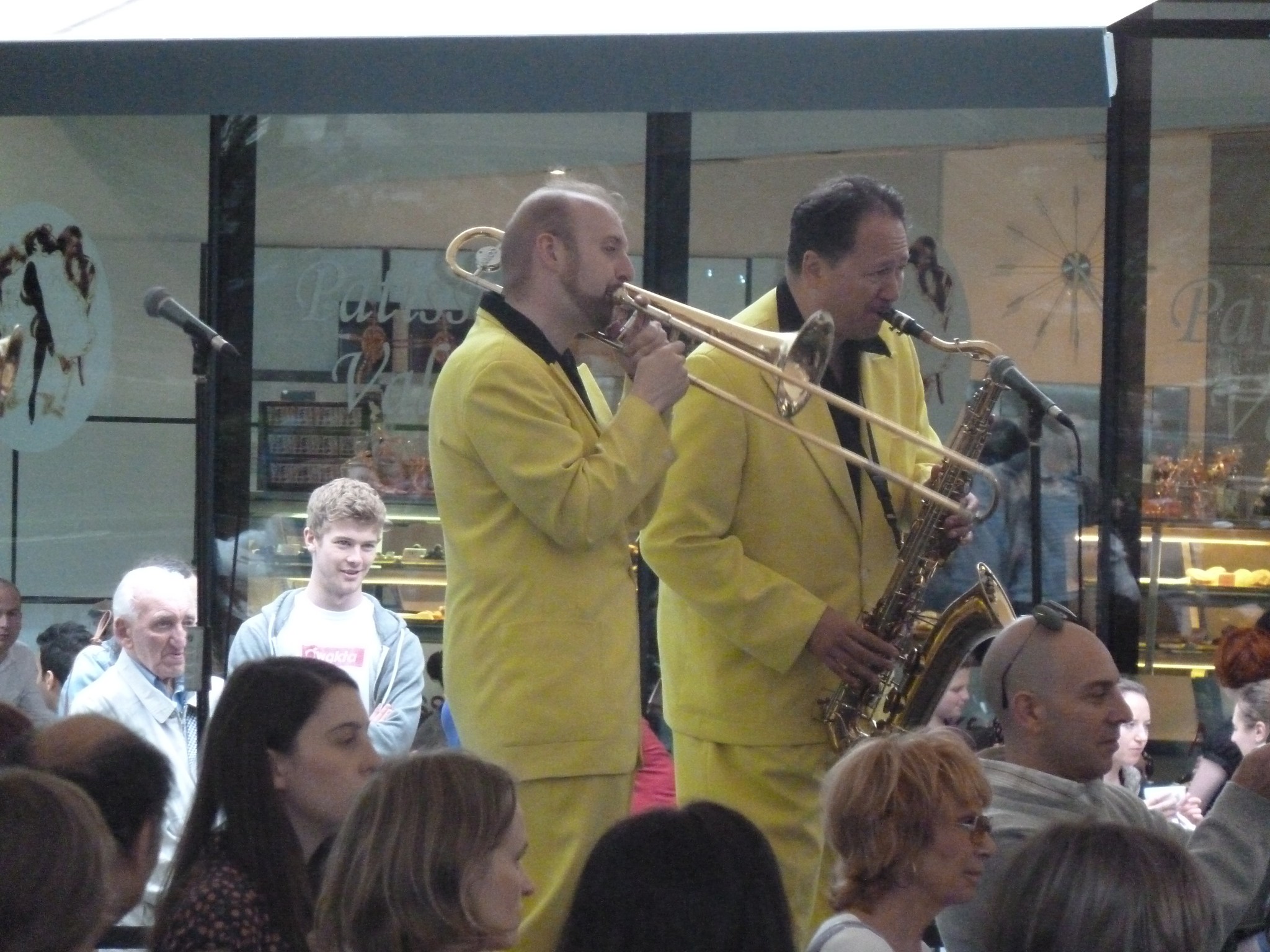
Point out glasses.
[931,815,995,844]
[1001,600,1079,716]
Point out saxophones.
[810,305,1018,757]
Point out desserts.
[401,543,429,562]
[1186,567,1270,586]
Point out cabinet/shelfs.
[1067,449,1269,754]
[259,512,447,720]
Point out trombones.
[439,224,1003,524]
[0,322,24,412]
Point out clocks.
[990,187,1156,353]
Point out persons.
[1099,679,1204,828]
[554,799,796,952]
[151,653,382,952]
[31,718,175,924]
[67,565,200,949]
[0,576,52,734]
[641,176,983,952]
[1,702,36,776]
[1230,679,1270,759]
[430,188,687,952]
[994,823,1223,950]
[58,554,200,723]
[981,398,1088,614]
[2,767,120,951]
[317,752,532,952]
[1189,626,1269,813]
[36,621,95,715]
[926,656,970,733]
[232,476,425,765]
[1108,488,1141,671]
[804,725,993,952]
[936,617,1270,951]
